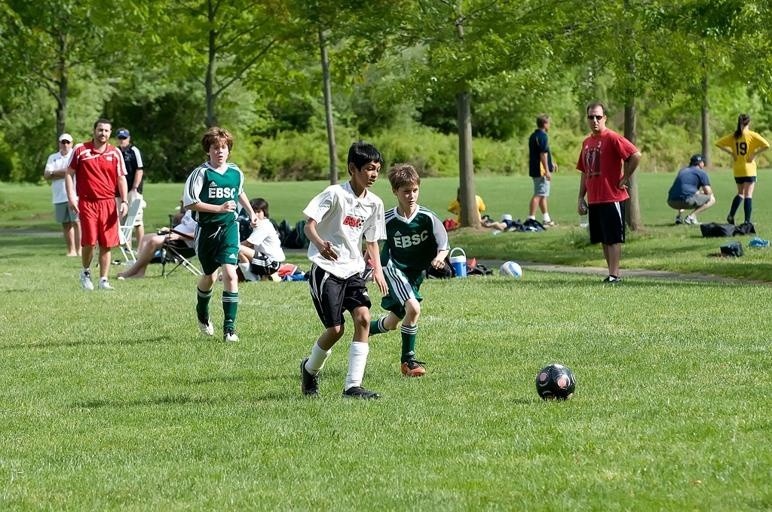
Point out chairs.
[94,198,142,269]
[161,221,204,277]
[168,199,197,228]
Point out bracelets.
[49,170,53,175]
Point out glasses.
[587,113,604,121]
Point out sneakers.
[674,215,683,225]
[299,357,320,395]
[542,220,559,229]
[341,384,380,401]
[400,350,426,378]
[195,303,214,337]
[726,215,735,225]
[684,213,699,225]
[124,247,140,255]
[79,269,96,291]
[223,323,239,343]
[602,275,621,282]
[98,276,116,290]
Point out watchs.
[120,200,129,205]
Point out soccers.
[500,261,522,281]
[537,365,576,400]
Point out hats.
[59,133,73,143]
[690,153,706,163]
[115,127,130,139]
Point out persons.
[298,143,390,399]
[115,127,145,255]
[667,153,715,225]
[43,133,83,258]
[527,112,560,227]
[447,187,486,228]
[117,208,197,279]
[575,102,642,284]
[238,197,286,282]
[367,163,452,376]
[714,113,770,227]
[64,119,130,290]
[181,126,260,343]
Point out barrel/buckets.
[503,213,512,226]
[449,247,467,277]
